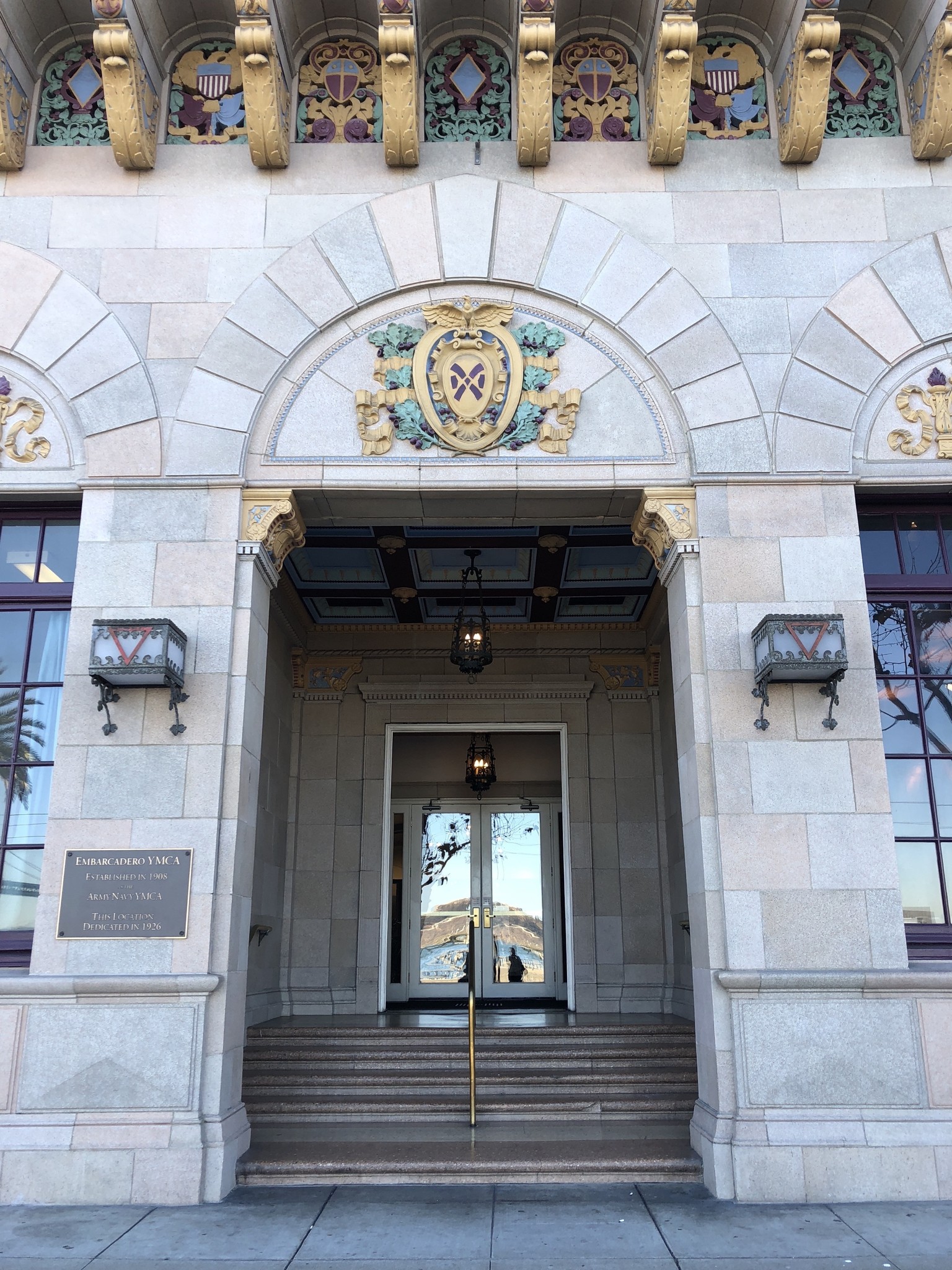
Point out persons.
[506,947,525,982]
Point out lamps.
[88,618,190,736]
[450,549,493,684]
[465,733,497,800]
[751,613,848,732]
